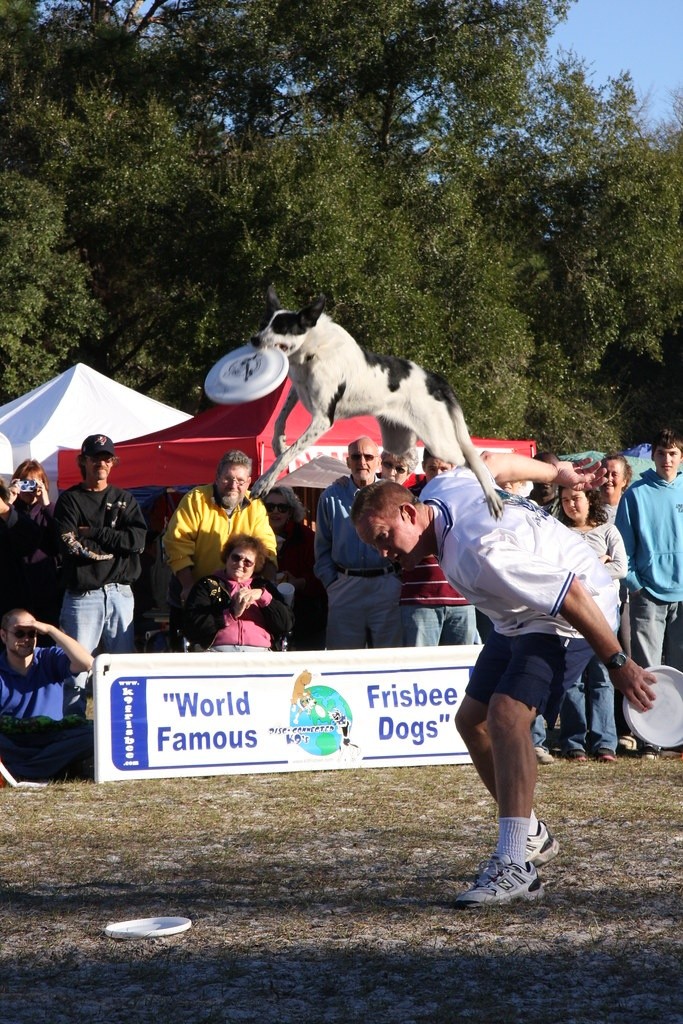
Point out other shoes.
[642,741,662,760]
[599,755,615,762]
[535,746,554,765]
[574,756,586,762]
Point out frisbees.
[622,664,683,748]
[204,343,290,406]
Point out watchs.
[604,650,628,670]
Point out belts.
[339,566,396,577]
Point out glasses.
[7,629,36,639]
[350,453,377,462]
[265,502,292,513]
[220,476,245,486]
[383,460,406,475]
[231,552,254,568]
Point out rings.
[181,600,185,603]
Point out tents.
[0,363,538,493]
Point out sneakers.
[525,820,559,865]
[456,854,545,905]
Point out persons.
[56,434,147,656]
[519,428,683,764]
[0,460,63,647]
[162,436,527,654]
[0,608,95,788]
[351,451,657,908]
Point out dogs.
[249,285,506,523]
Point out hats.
[81,435,114,456]
